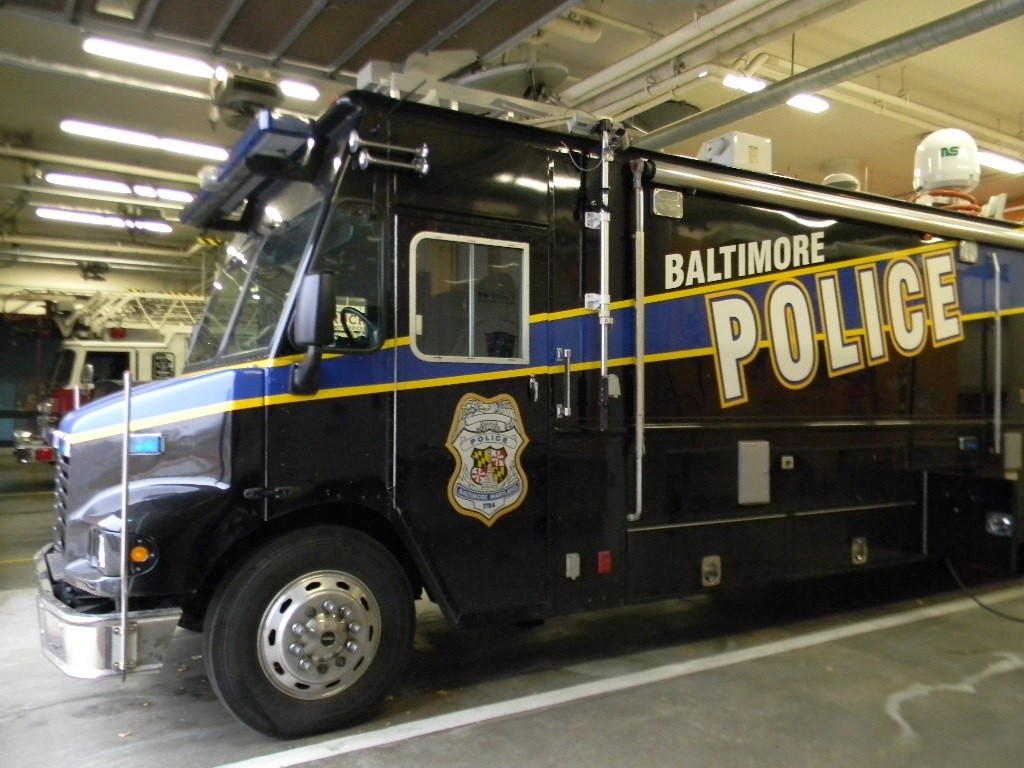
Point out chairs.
[425,275,519,356]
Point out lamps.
[722,75,829,115]
[34,35,321,233]
[977,149,1024,175]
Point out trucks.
[11,47,1024,742]
